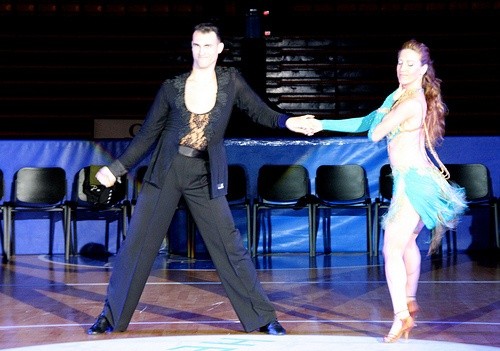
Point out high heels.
[408,300,421,318]
[385,315,413,343]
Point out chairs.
[0,163,499,269]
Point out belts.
[179,145,208,159]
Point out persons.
[87,23,314,336]
[301,40,470,342]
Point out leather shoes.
[259,319,286,334]
[88,314,110,334]
